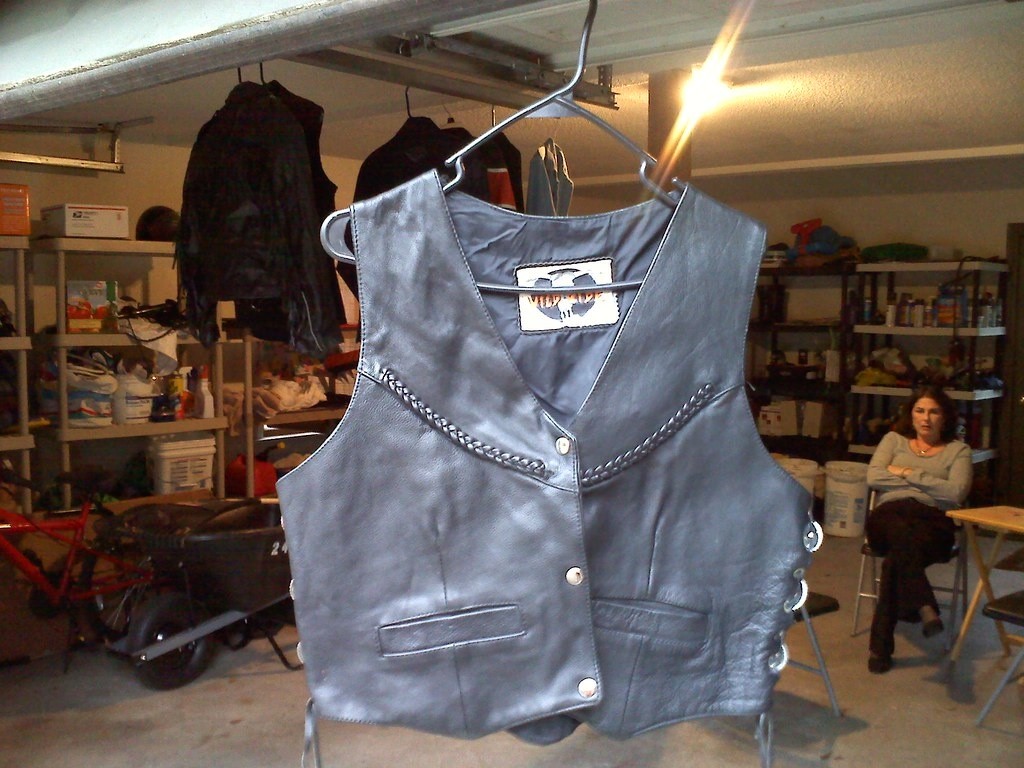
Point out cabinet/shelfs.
[0,235,1005,514]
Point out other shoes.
[923,618,944,639]
[868,652,891,672]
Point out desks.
[943,506,1024,678]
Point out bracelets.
[901,467,910,476]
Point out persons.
[866,387,974,671]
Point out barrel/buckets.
[146,431,216,495]
[111,373,153,425]
[768,454,872,538]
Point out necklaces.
[915,444,932,455]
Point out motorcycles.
[106,489,305,688]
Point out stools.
[971,591,1024,725]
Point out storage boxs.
[786,275,842,324]
[39,203,129,239]
[0,183,31,235]
[65,281,120,335]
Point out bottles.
[748,269,1005,452]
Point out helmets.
[136,206,180,241]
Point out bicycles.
[0,469,218,640]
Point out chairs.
[851,487,968,651]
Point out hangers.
[203,0,766,294]
